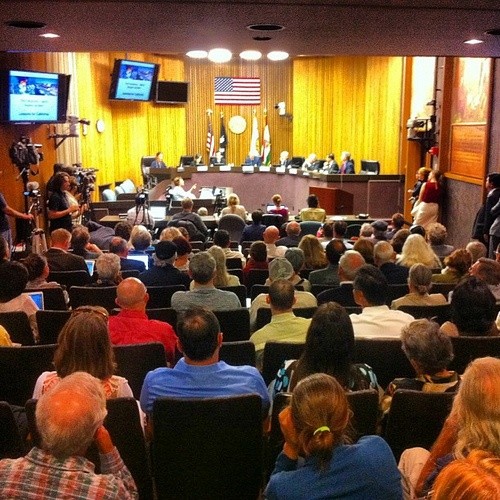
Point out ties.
[341,164,344,174]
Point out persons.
[33,311,134,399]
[471,172,500,258]
[408,167,442,226]
[273,302,382,394]
[169,177,197,201]
[397,356,500,500]
[0,371,139,500]
[0,194,500,347]
[119,65,154,80]
[431,449,500,500]
[140,307,271,413]
[46,163,89,236]
[263,373,403,500]
[9,79,59,96]
[151,152,355,175]
[382,320,462,411]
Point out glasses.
[70,307,108,326]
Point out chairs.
[141,157,154,185]
[290,157,305,168]
[180,157,194,167]
[0,214,500,500]
[102,179,137,202]
[361,161,380,175]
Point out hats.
[371,220,388,231]
[269,258,293,281]
[285,247,304,267]
[156,241,178,260]
[213,230,230,242]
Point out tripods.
[76,185,95,226]
[212,195,224,217]
[134,200,152,230]
[27,198,49,254]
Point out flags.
[205,109,214,158]
[261,124,272,166]
[220,115,227,163]
[214,77,261,105]
[249,117,261,166]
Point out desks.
[99,213,373,228]
[198,186,233,199]
[150,167,405,214]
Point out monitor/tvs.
[107,60,159,104]
[155,81,192,104]
[2,70,60,123]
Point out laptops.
[84,259,95,276]
[127,254,150,271]
[22,291,45,311]
